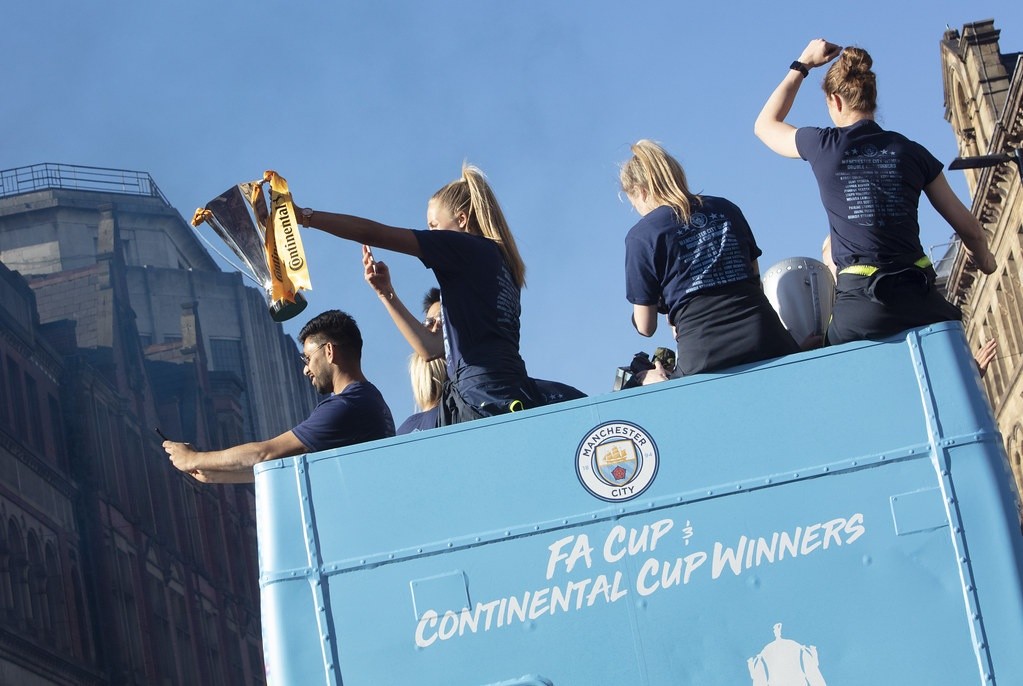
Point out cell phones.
[366,245,379,274]
[156,428,167,440]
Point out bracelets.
[378,288,394,300]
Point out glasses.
[421,316,442,329]
[299,344,336,365]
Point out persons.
[397,286,589,434]
[162,311,397,484]
[754,39,997,347]
[267,164,538,427]
[618,139,801,389]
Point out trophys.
[192,169,308,322]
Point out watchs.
[301,208,313,227]
[789,60,809,78]
